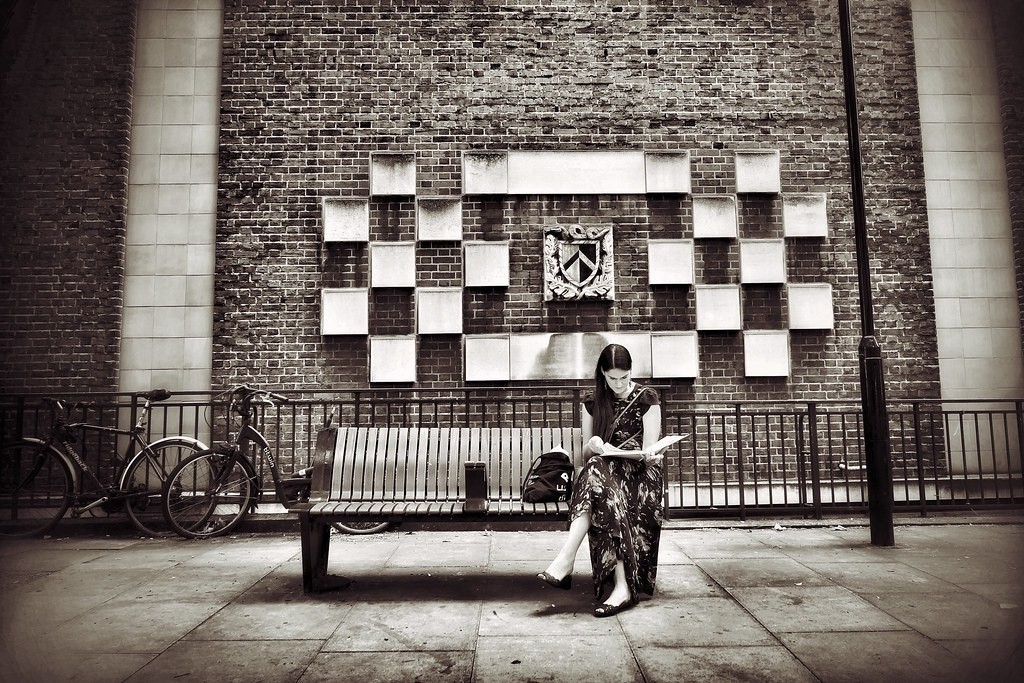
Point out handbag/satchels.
[523,452,574,504]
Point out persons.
[536,344,664,617]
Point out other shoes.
[594,594,635,618]
[536,571,572,590]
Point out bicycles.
[162,382,396,539]
[1,388,218,539]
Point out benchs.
[287,416,583,594]
